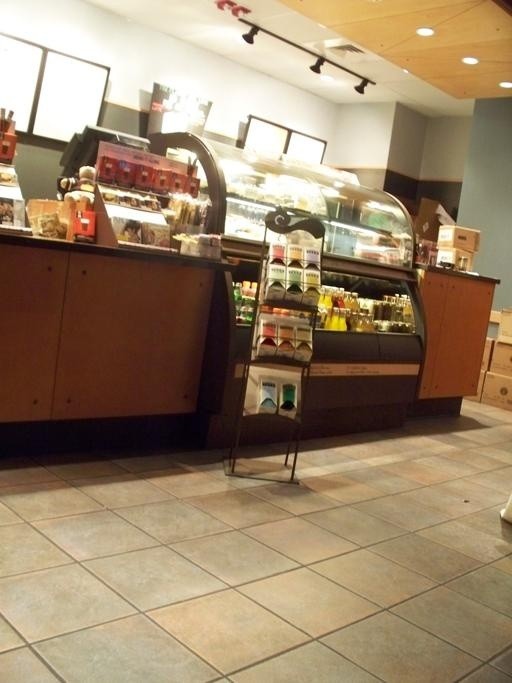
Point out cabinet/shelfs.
[197,251,426,450]
[148,130,423,284]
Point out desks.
[0,229,232,435]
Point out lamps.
[354,79,368,97]
[310,58,326,73]
[243,27,259,45]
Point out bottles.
[232,281,257,324]
[458,257,468,272]
[315,285,415,333]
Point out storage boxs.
[434,248,474,272]
[463,371,485,403]
[489,341,512,376]
[436,224,481,252]
[498,309,512,343]
[489,310,502,323]
[480,338,493,372]
[480,371,512,412]
